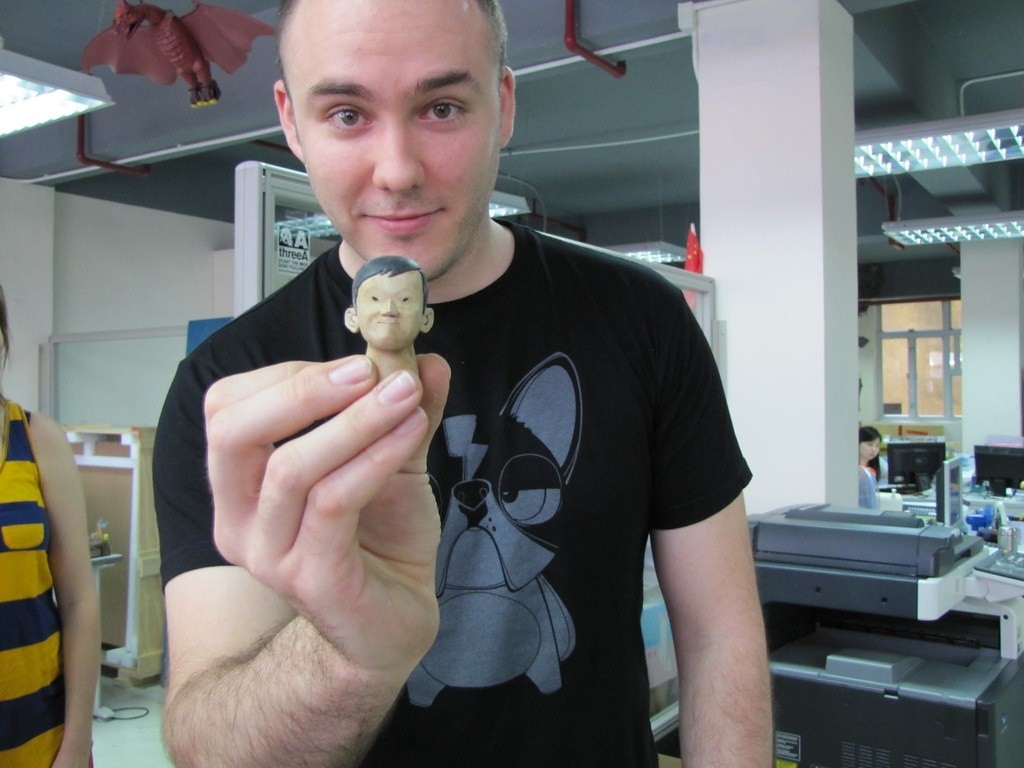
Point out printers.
[744,500,1024,768]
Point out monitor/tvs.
[887,442,1024,527]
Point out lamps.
[0,49,115,140]
[881,209,1024,246]
[853,107,1024,179]
[607,239,686,264]
[489,190,531,219]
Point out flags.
[684,225,704,308]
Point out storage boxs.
[642,563,681,718]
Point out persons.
[152,0,773,768]
[0,284,100,768]
[859,426,882,479]
[343,255,434,382]
